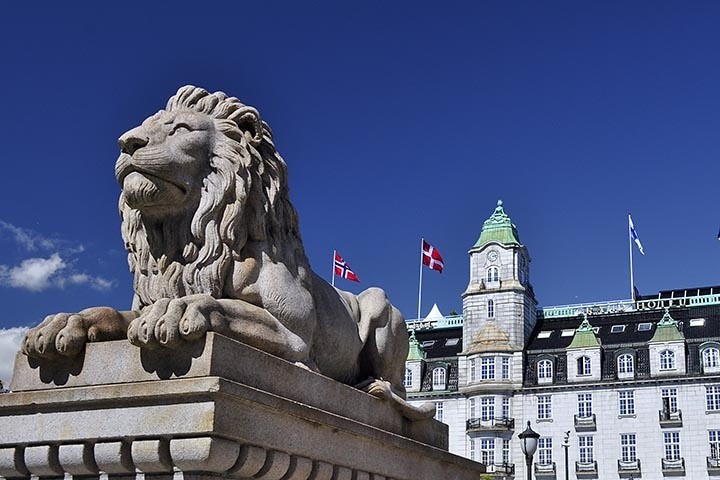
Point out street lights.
[517,419,541,480]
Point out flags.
[630,218,645,256]
[423,239,444,273]
[334,252,361,283]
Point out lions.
[21,85,437,417]
